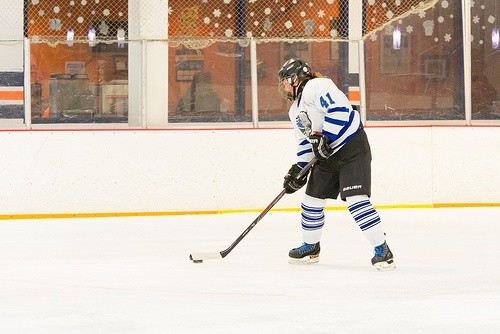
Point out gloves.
[283,164,309,194]
[308,132,333,162]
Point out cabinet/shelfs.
[49,79,128,117]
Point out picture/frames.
[378,32,413,76]
[419,56,450,83]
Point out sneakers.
[289,241,320,264]
[371,240,396,271]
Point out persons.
[277,58,397,272]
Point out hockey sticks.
[188,155,317,260]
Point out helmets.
[276,58,312,101]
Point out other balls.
[192,260,203,263]
